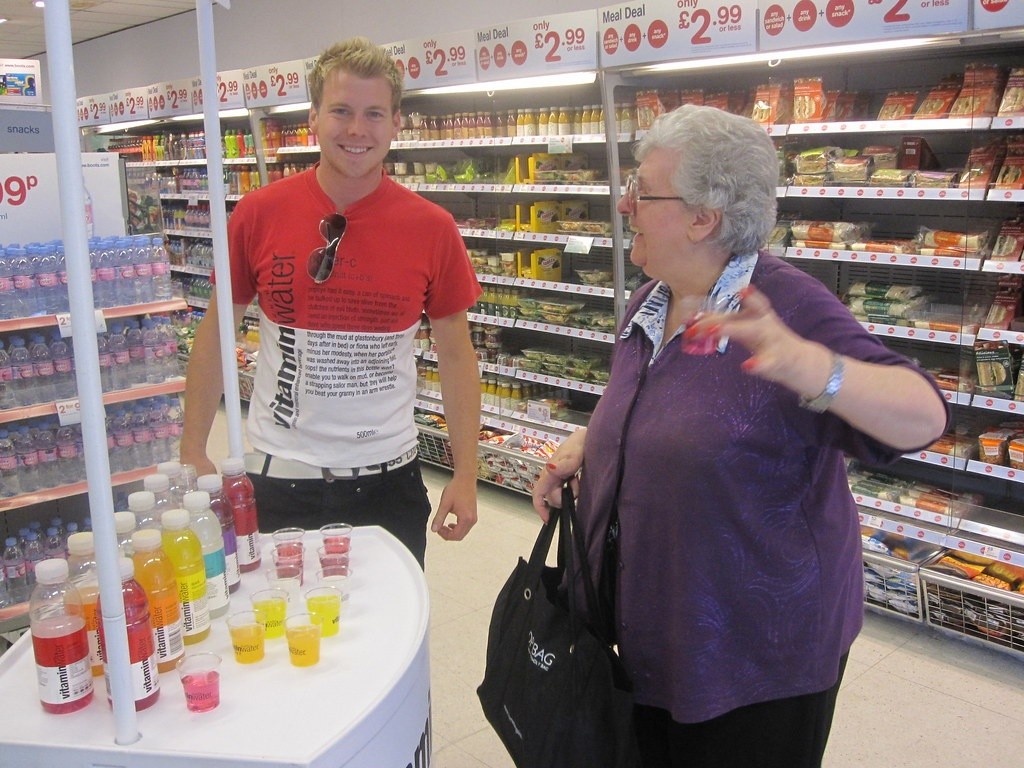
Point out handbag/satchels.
[475,491,641,768]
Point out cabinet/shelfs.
[278,116,1024,567]
[0,297,188,623]
[127,157,276,319]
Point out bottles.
[29,558,94,714]
[220,457,261,573]
[183,491,230,618]
[0,235,198,623]
[160,510,211,644]
[67,532,104,676]
[197,474,242,594]
[97,557,160,711]
[108,96,636,412]
[131,529,186,673]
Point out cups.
[272,527,305,549]
[317,543,351,576]
[320,523,352,542]
[176,652,222,712]
[271,544,305,588]
[304,587,340,638]
[266,565,302,606]
[226,610,267,664]
[251,588,289,640]
[315,565,352,613]
[284,613,322,667]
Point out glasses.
[625,175,683,208]
[306,214,347,285]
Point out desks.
[0,522,430,768]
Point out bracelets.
[798,348,844,414]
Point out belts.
[245,446,418,480]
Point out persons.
[181,38,481,573]
[533,104,953,768]
